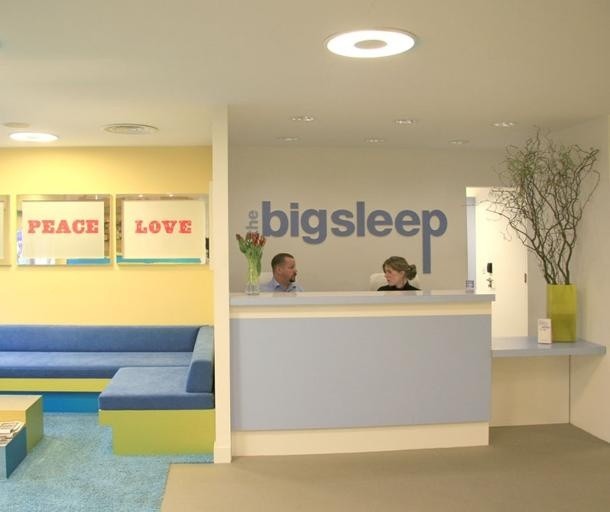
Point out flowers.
[235,230,266,284]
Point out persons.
[258,253,305,291]
[377,256,419,291]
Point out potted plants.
[471,127,603,344]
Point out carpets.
[1,409,214,511]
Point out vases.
[244,272,261,296]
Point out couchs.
[0,324,216,456]
